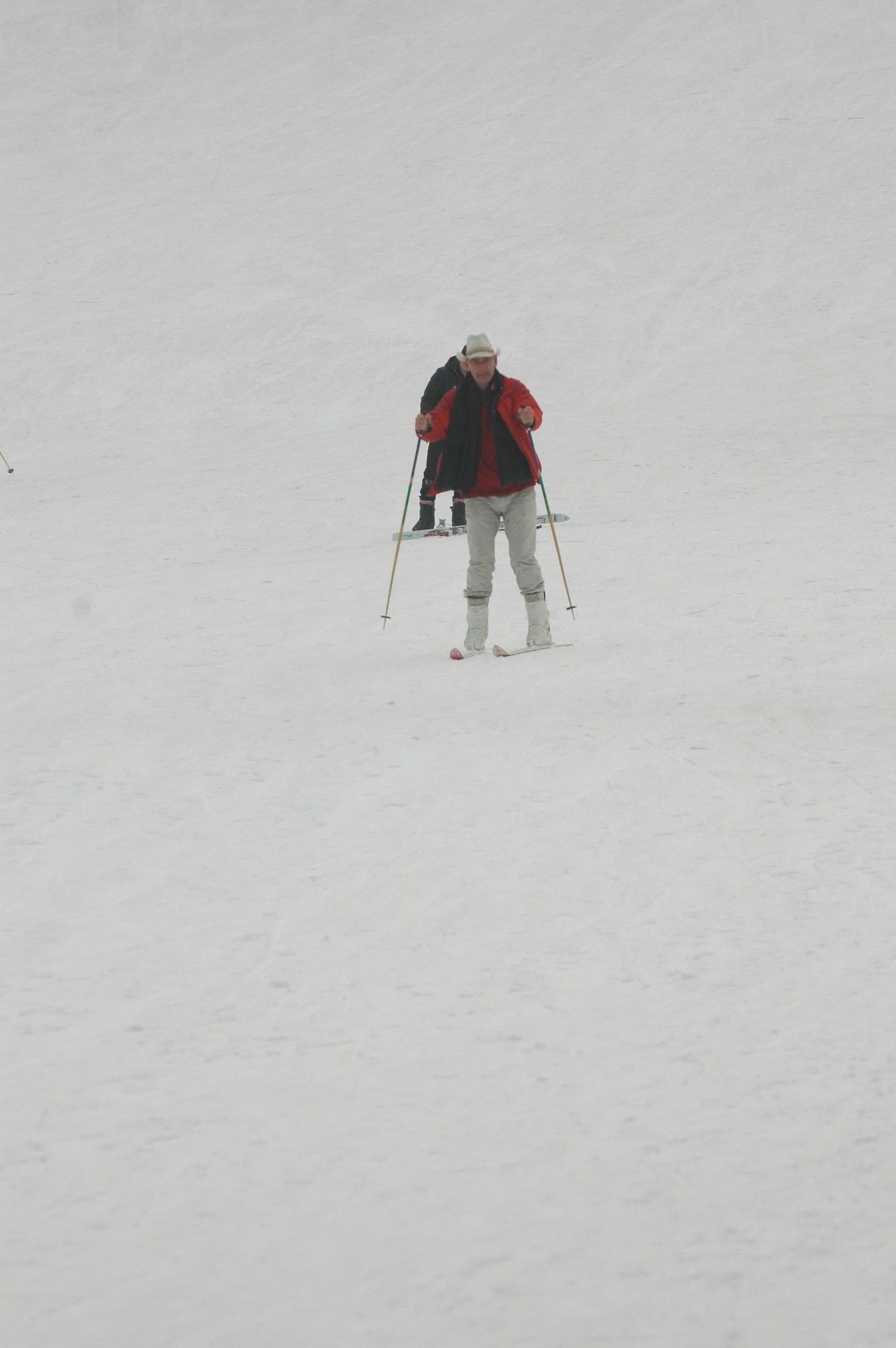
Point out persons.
[411,345,468,532]
[414,333,553,650]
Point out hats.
[464,333,501,359]
[457,347,468,362]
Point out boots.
[451,495,467,526]
[412,497,435,531]
[524,592,552,645]
[464,595,489,650]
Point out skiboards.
[394,514,570,541]
[450,642,573,659]
